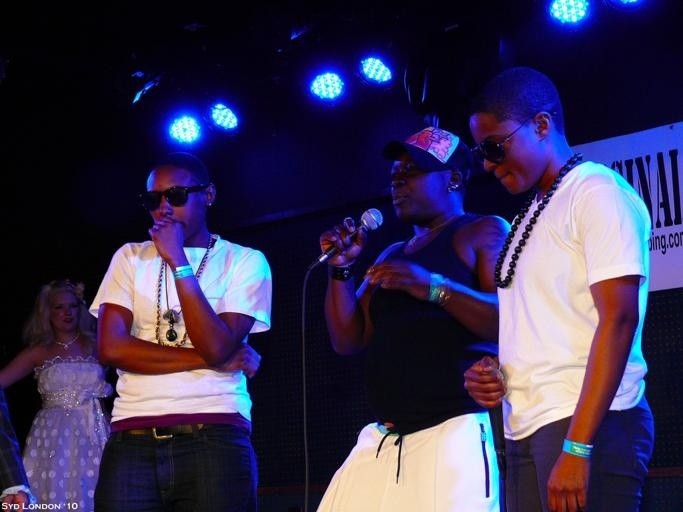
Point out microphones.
[317,208,383,263]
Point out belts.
[124,425,230,436]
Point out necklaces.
[162,263,184,341]
[155,232,214,350]
[57,335,81,351]
[495,150,584,287]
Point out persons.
[315,125,511,512]
[0,275,115,512]
[461,64,656,512]
[88,154,271,512]
[0,381,36,512]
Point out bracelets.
[171,265,194,280]
[561,439,593,461]
[429,267,451,307]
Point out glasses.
[473,125,531,163]
[140,185,189,210]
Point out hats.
[384,126,472,173]
